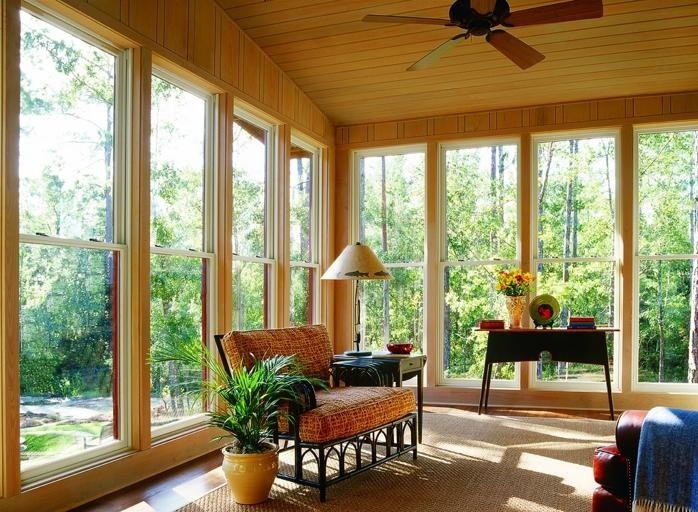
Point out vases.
[505,296,527,329]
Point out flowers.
[495,268,536,295]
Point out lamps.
[321,242,394,356]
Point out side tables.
[334,360,393,444]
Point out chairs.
[592,409,697,512]
[214,324,418,502]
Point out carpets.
[174,407,618,512]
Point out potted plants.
[145,334,331,504]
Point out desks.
[474,327,620,421]
[335,352,427,451]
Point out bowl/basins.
[386,343,413,354]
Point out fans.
[362,0,603,71]
